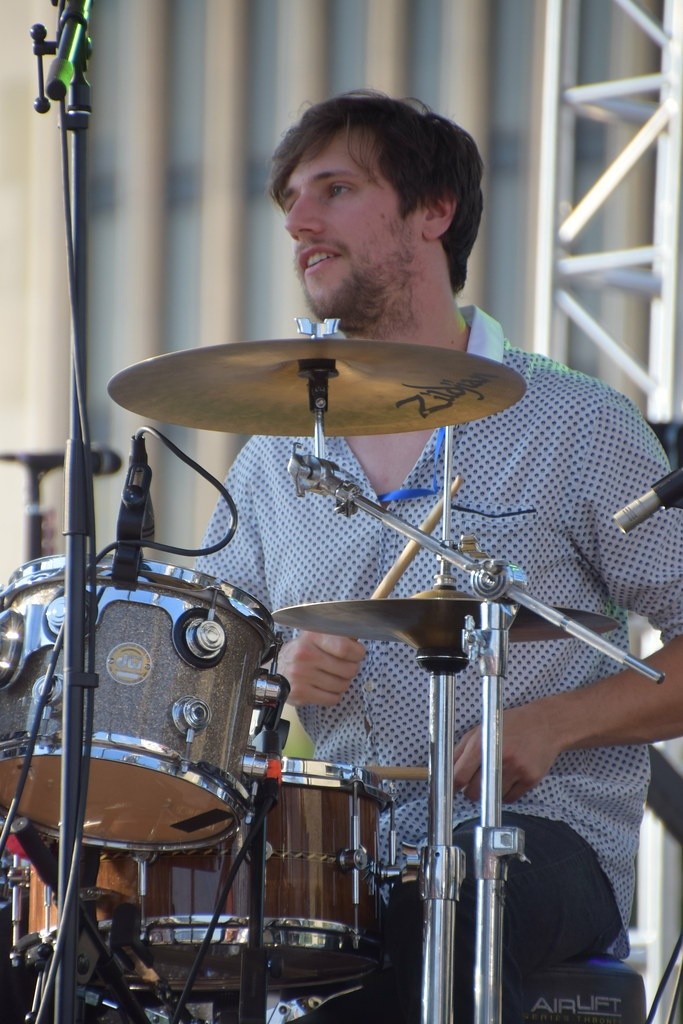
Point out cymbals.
[267,590,619,648]
[105,335,528,441]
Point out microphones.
[617,463,681,535]
[128,432,156,547]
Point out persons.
[204,88,681,1023]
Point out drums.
[24,755,393,990]
[0,553,277,855]
